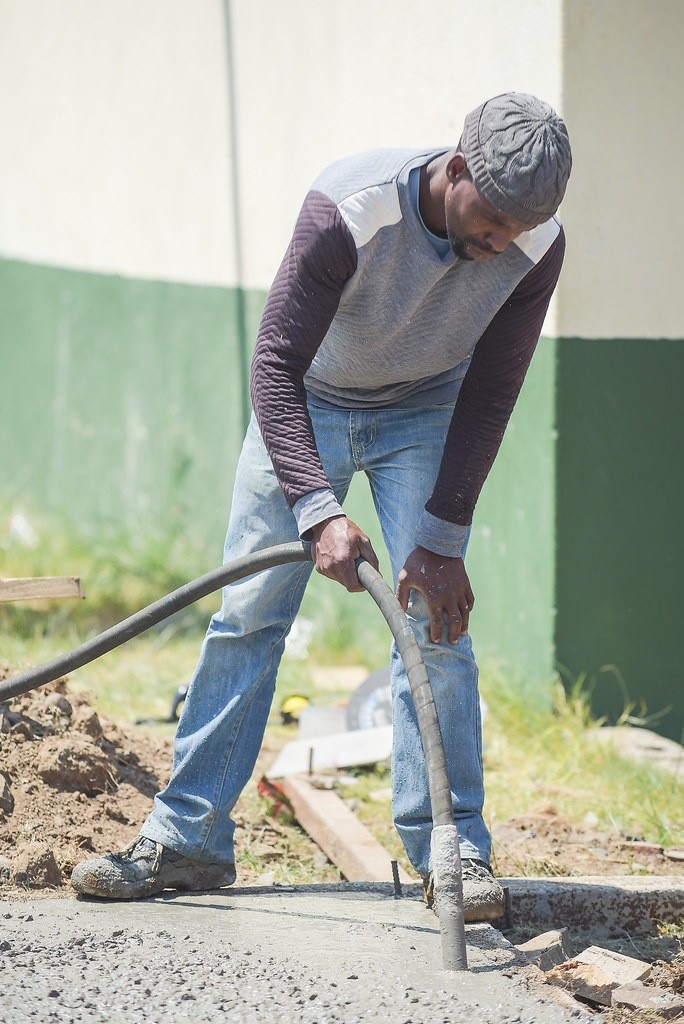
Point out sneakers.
[423,858,507,922]
[71,835,236,899]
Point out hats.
[462,91,573,225]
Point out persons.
[70,90,572,921]
